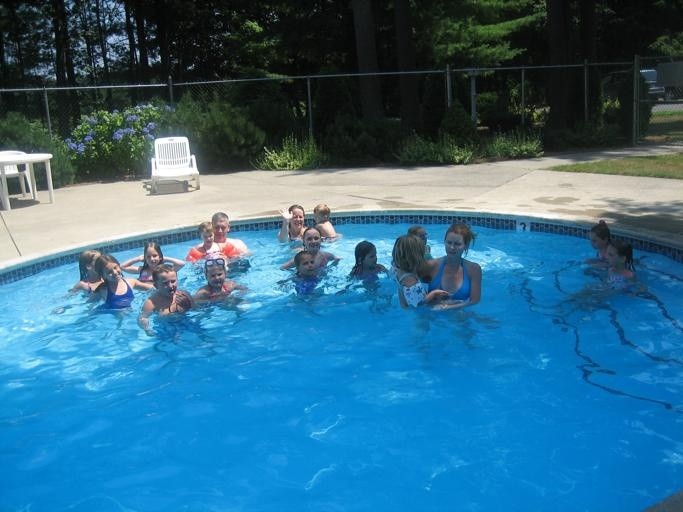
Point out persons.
[119,242,183,286]
[277,204,309,244]
[390,234,449,309]
[313,203,341,242]
[348,240,385,275]
[137,264,196,337]
[211,212,251,258]
[417,223,483,309]
[603,239,636,280]
[185,222,224,260]
[194,258,248,304]
[70,249,104,294]
[85,254,156,312]
[407,226,435,261]
[287,251,316,276]
[280,227,342,269]
[587,219,612,262]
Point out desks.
[1,153,53,208]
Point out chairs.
[141,137,201,194]
[0,151,33,200]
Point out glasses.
[205,259,225,266]
